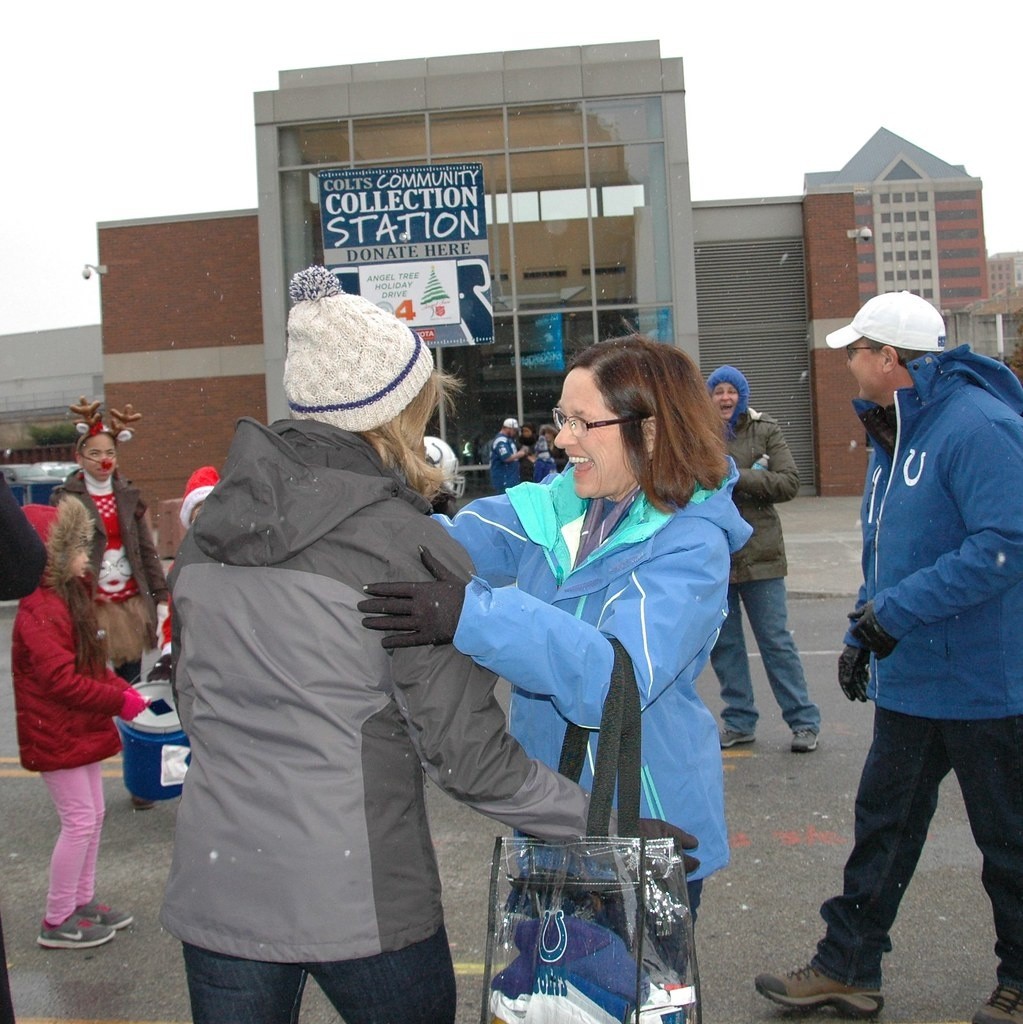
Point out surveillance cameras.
[860,228,872,241]
[82,268,91,280]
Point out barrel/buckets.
[114,665,197,800]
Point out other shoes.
[130,793,157,809]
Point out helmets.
[421,436,465,497]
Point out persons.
[157,268,702,1022]
[357,331,753,1023]
[145,466,225,684]
[1,470,47,1024]
[42,397,171,813]
[488,418,568,495]
[705,364,827,753]
[752,290,1023,1024]
[418,436,465,522]
[12,497,160,950]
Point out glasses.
[553,407,651,437]
[846,344,883,361]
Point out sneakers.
[754,962,885,1017]
[790,728,820,752]
[971,979,1023,1024]
[37,916,116,949]
[74,895,134,932]
[719,728,756,747]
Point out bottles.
[737,454,770,499]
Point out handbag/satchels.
[481,635,703,1024]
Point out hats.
[180,466,223,530]
[825,290,946,352]
[503,417,522,431]
[283,265,434,432]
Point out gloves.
[848,601,899,660]
[637,817,699,875]
[357,544,469,648]
[117,686,152,722]
[837,645,875,703]
[146,654,176,682]
